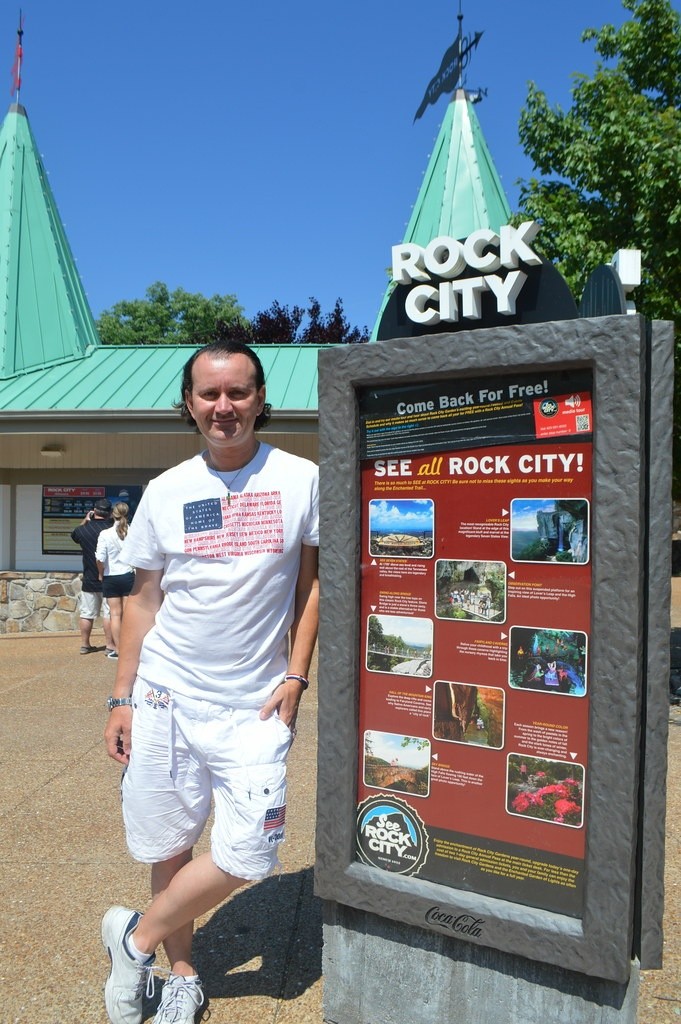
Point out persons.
[520,761,528,786]
[71,498,137,660]
[447,591,492,616]
[101,340,320,1023]
[476,716,485,730]
[517,646,561,656]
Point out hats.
[94,498,112,511]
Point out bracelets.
[284,674,309,690]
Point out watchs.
[107,696,132,711]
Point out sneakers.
[101,906,176,1024]
[104,649,114,656]
[108,651,119,658]
[152,972,208,1024]
[81,646,99,654]
